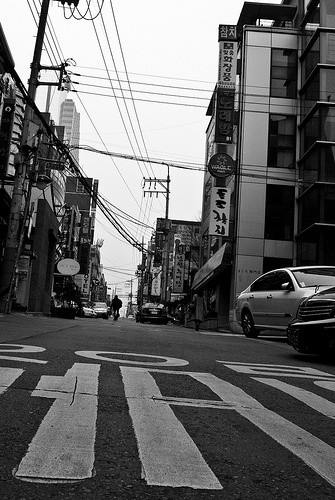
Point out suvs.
[128,302,168,325]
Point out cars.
[235,266,335,365]
[56,299,110,319]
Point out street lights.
[3,174,53,314]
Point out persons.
[111,295,120,320]
[192,290,209,331]
